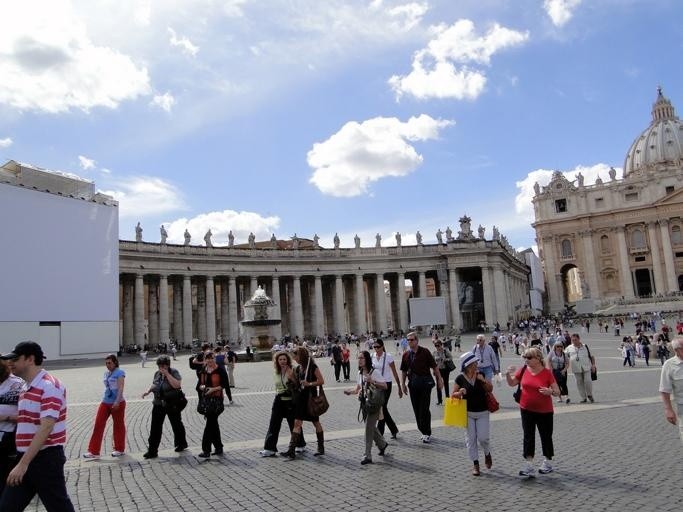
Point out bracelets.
[4,416,10,421]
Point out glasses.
[522,355,533,360]
[556,345,563,348]
[434,344,441,348]
[373,345,382,349]
[407,339,415,341]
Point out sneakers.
[472,453,554,477]
[558,396,595,404]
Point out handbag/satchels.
[419,372,435,390]
[513,389,522,403]
[197,394,224,416]
[487,391,499,413]
[161,387,187,415]
[443,392,468,428]
[309,387,329,417]
[444,360,456,372]
[272,391,295,418]
[364,382,385,414]
[591,366,597,381]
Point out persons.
[0,353,28,494]
[451,310,594,404]
[83,354,128,459]
[0,340,76,512]
[533,181,540,195]
[195,350,229,457]
[186,338,237,404]
[140,355,188,459]
[343,324,464,465]
[575,172,584,186]
[245,333,361,457]
[120,337,178,369]
[608,166,616,180]
[598,309,682,367]
[505,347,562,476]
[659,338,682,435]
[452,351,492,475]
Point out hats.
[1,342,47,360]
[459,352,480,372]
